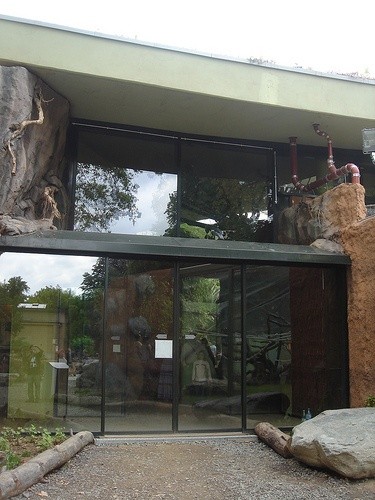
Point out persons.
[26,344,44,402]
